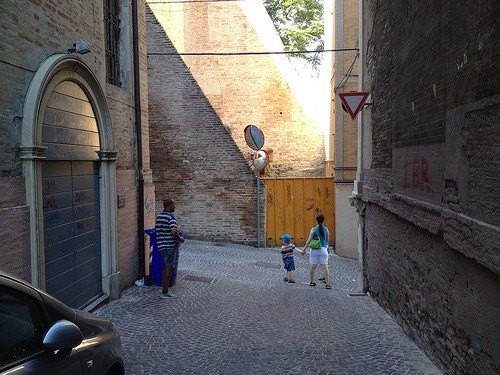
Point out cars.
[0,269,125,375]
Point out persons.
[155,198,185,298]
[281,234,305,282]
[302,213,332,289]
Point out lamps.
[69,41,91,55]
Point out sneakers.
[160,292,176,298]
[158,288,163,292]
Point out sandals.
[310,282,316,286]
[326,285,331,289]
[288,279,295,283]
[284,277,288,282]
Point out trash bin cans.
[144,227,187,287]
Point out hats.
[282,234,291,243]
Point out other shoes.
[323,280,326,283]
[317,277,325,280]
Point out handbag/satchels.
[309,227,321,249]
